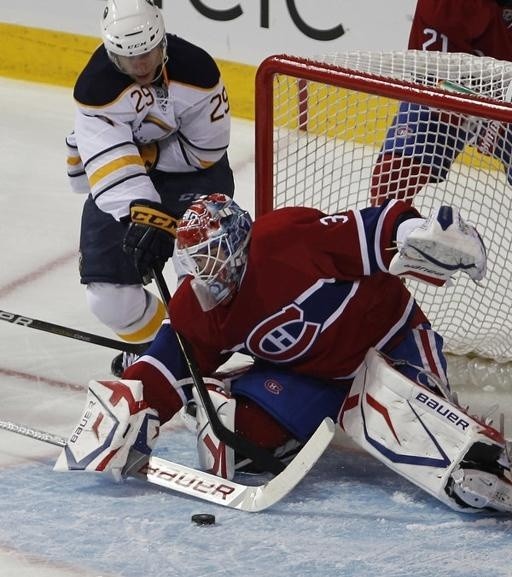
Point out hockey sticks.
[151,266,286,478]
[1,417,336,513]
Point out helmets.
[100,0,168,74]
[175,192,253,312]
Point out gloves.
[121,200,180,277]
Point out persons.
[368,0,512,210]
[65,0,235,379]
[54,194,512,520]
